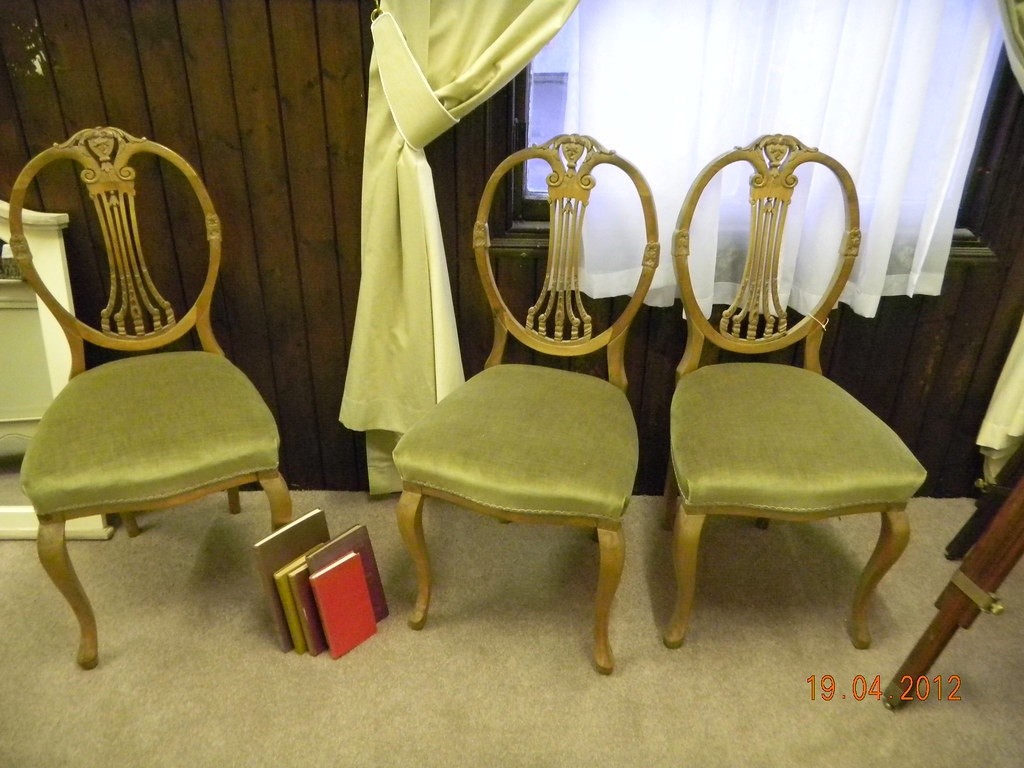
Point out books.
[255,504,388,661]
[310,552,379,659]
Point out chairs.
[392,132,664,674]
[669,133,926,651]
[9,127,291,670]
